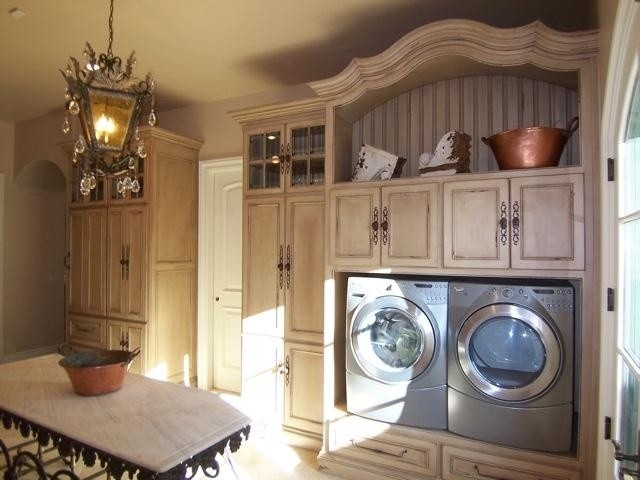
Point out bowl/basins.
[477,117,578,170]
[57,344,145,397]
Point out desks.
[1,353,251,480]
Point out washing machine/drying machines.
[345,276,449,432]
[448,282,574,454]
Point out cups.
[293,173,323,183]
[294,134,324,155]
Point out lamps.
[60,1,160,195]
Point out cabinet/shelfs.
[241,345,346,451]
[437,437,586,479]
[65,323,198,386]
[66,206,196,323]
[328,178,442,276]
[442,167,586,278]
[242,193,347,345]
[318,422,437,480]
[243,124,326,193]
[67,149,197,205]
